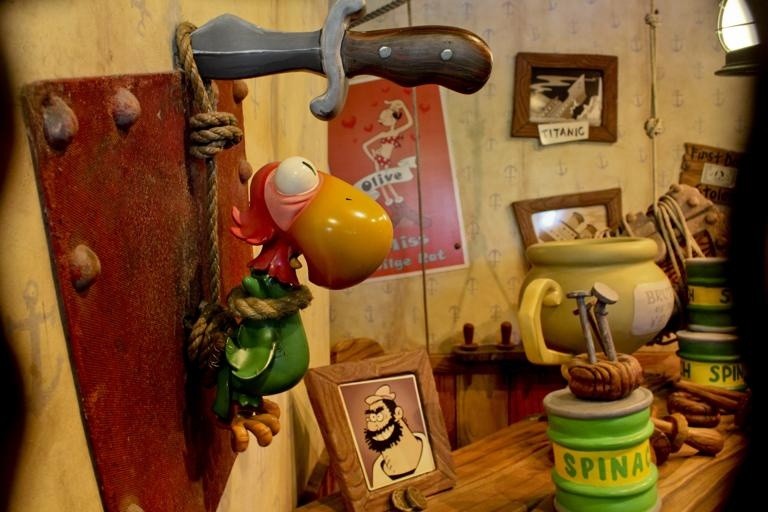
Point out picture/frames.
[510,47,619,145]
[305,346,456,512]
[511,188,624,247]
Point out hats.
[365,384,397,404]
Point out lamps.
[713,0,767,75]
